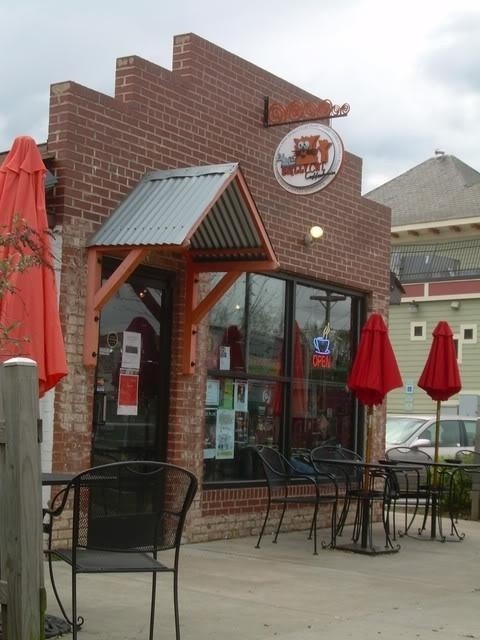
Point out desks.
[324,458,400,555]
[41,472,114,638]
[381,459,477,543]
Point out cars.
[386,414,480,497]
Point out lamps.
[303,226,326,246]
[406,300,419,312]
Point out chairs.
[306,445,363,546]
[387,447,435,540]
[454,450,480,542]
[254,446,338,556]
[69,462,199,638]
